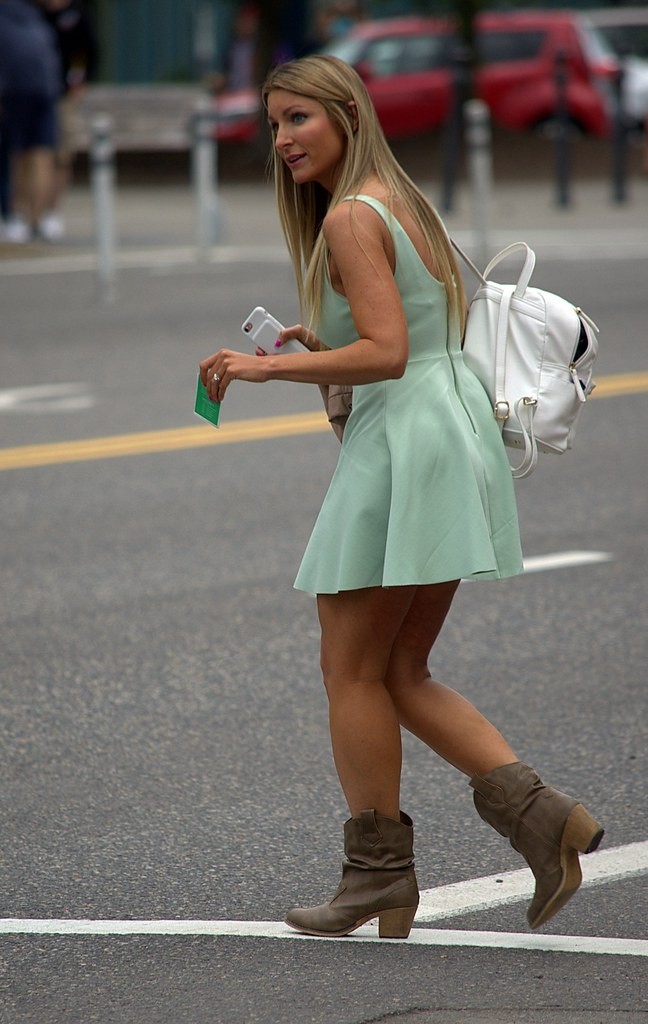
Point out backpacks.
[446,230,600,479]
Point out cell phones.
[242,306,311,355]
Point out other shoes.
[4,221,30,245]
[39,215,63,242]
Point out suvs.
[190,11,624,148]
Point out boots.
[468,761,604,930]
[284,809,420,937]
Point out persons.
[0,0,65,242]
[430,1,481,212]
[198,53,606,941]
[233,0,326,159]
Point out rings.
[213,373,220,382]
